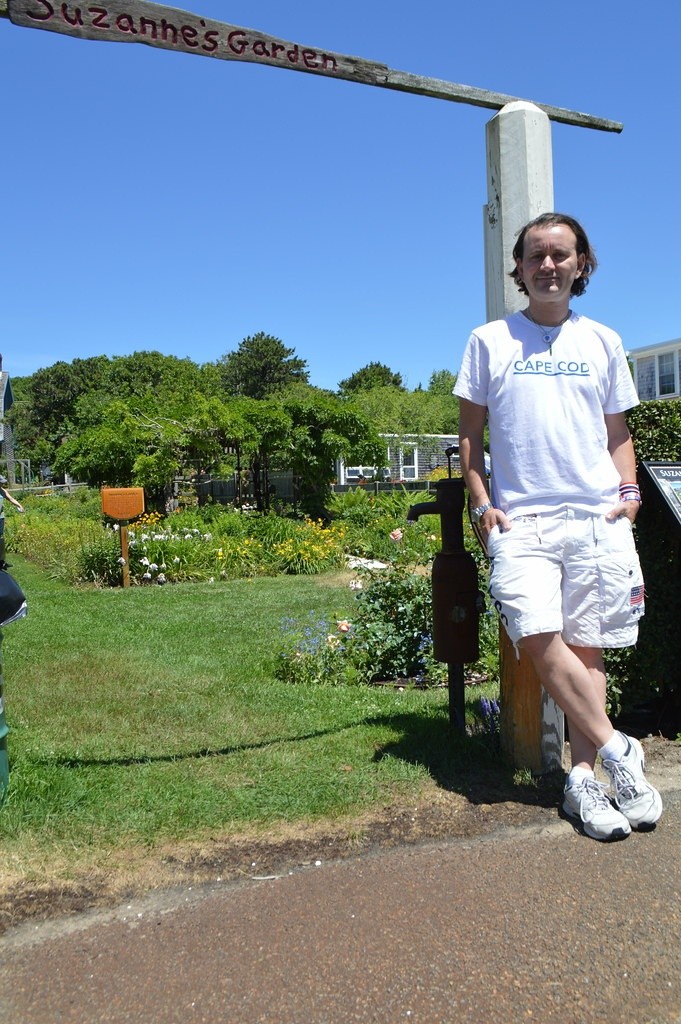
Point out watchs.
[471,503,494,523]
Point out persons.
[267,481,276,494]
[453,213,663,839]
[0,475,25,570]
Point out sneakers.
[594,732,664,831]
[562,774,632,842]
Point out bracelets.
[620,482,642,505]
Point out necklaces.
[526,307,570,356]
